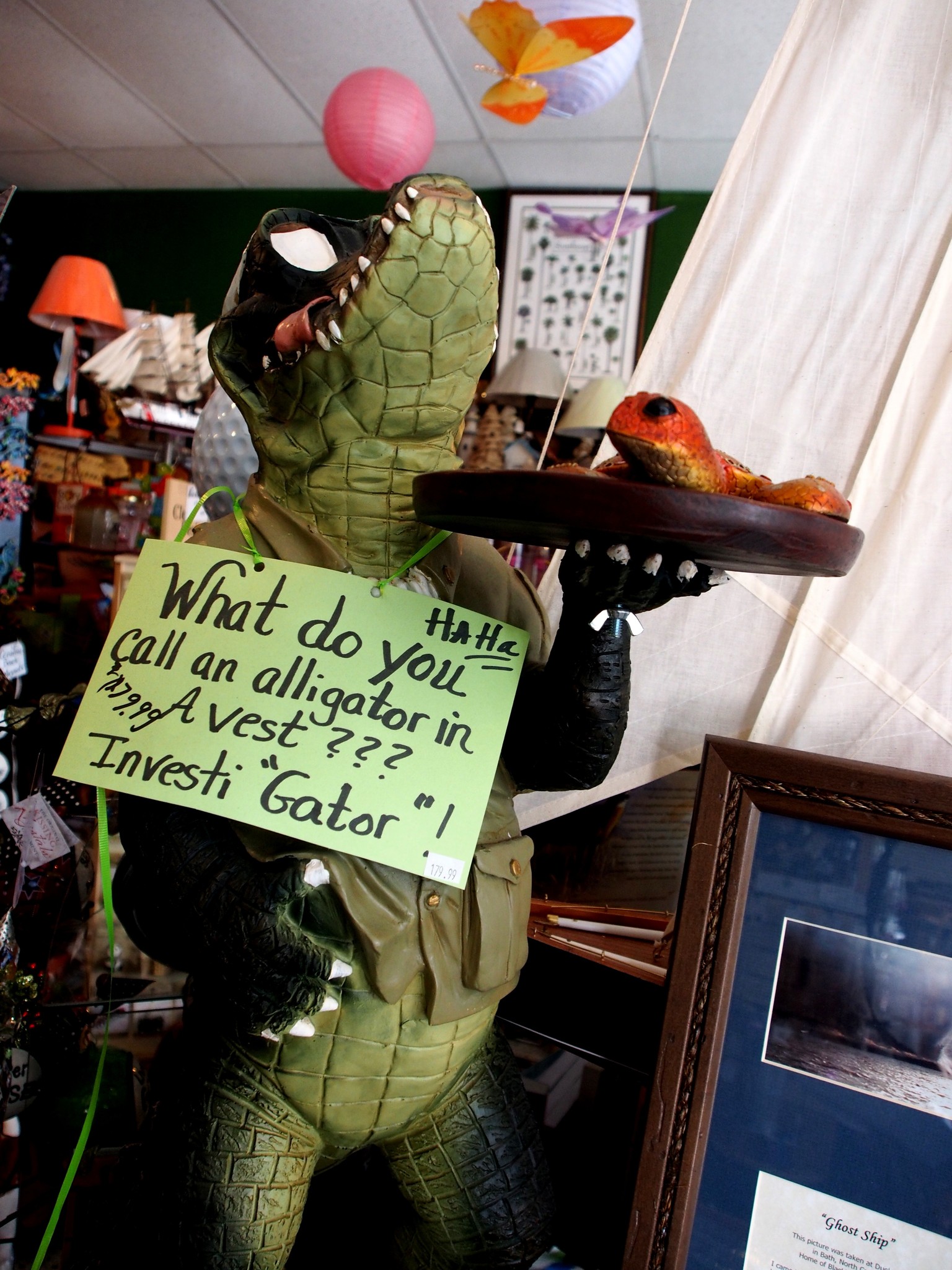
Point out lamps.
[26,251,122,437]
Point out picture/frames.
[498,186,658,404]
[627,733,952,1270]
